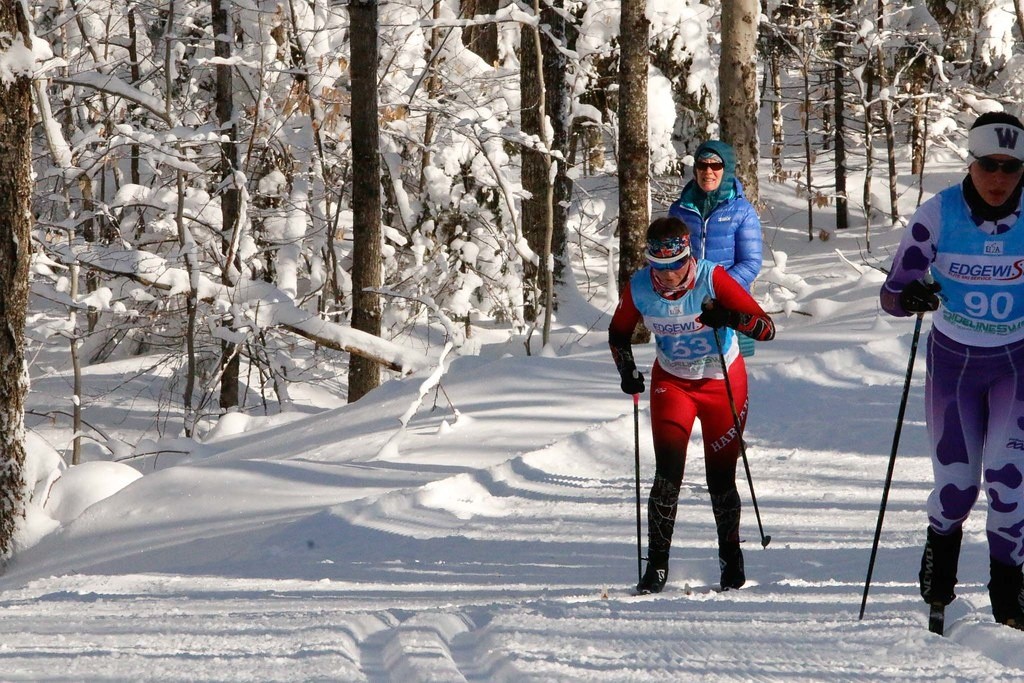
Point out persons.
[880,109,1024,632]
[608,218,774,596]
[669,142,762,356]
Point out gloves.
[901,279,942,313]
[699,300,740,330]
[617,361,645,395]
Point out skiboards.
[924,606,1024,636]
[593,578,768,598]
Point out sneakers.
[986,554,1024,631]
[637,549,669,593]
[719,541,745,590]
[919,525,963,606]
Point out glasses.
[694,162,725,171]
[645,234,693,271]
[968,149,1024,172]
